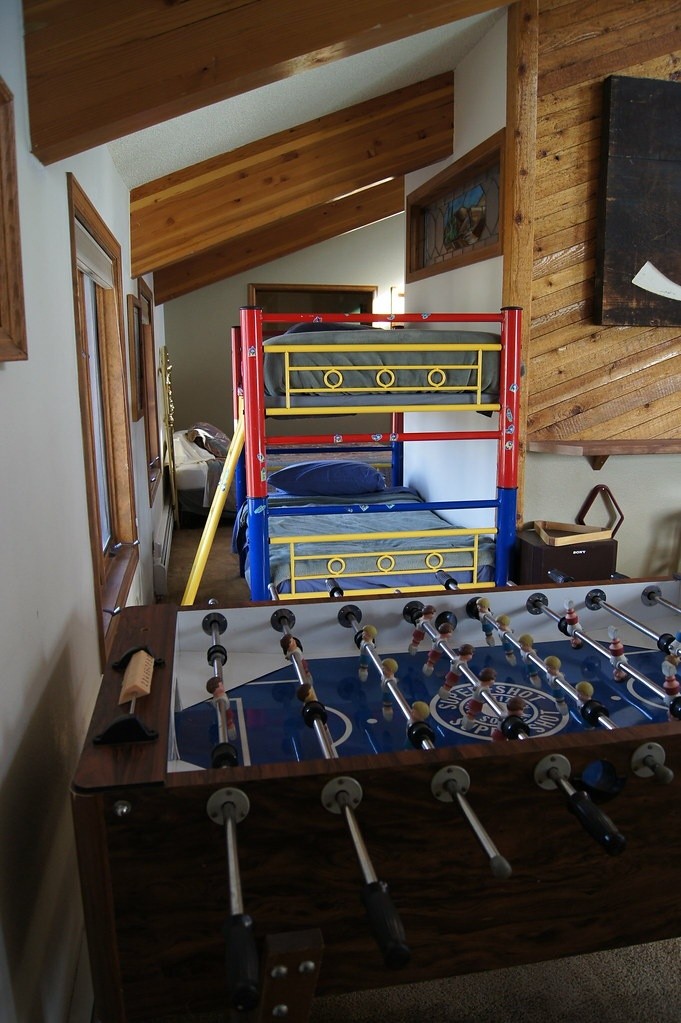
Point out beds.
[229,297,524,606]
[163,428,395,522]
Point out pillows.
[188,421,228,455]
[286,322,379,332]
[264,460,390,496]
